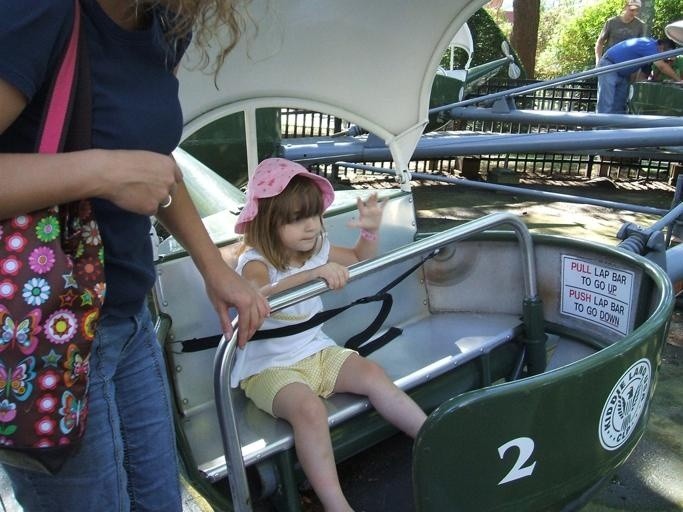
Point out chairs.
[146,190,524,488]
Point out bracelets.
[359,226,379,245]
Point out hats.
[234,158,335,234]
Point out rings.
[159,194,174,210]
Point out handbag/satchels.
[0,200,107,474]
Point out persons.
[594,0,646,82]
[594,37,682,113]
[224,154,431,512]
[1,1,272,512]
